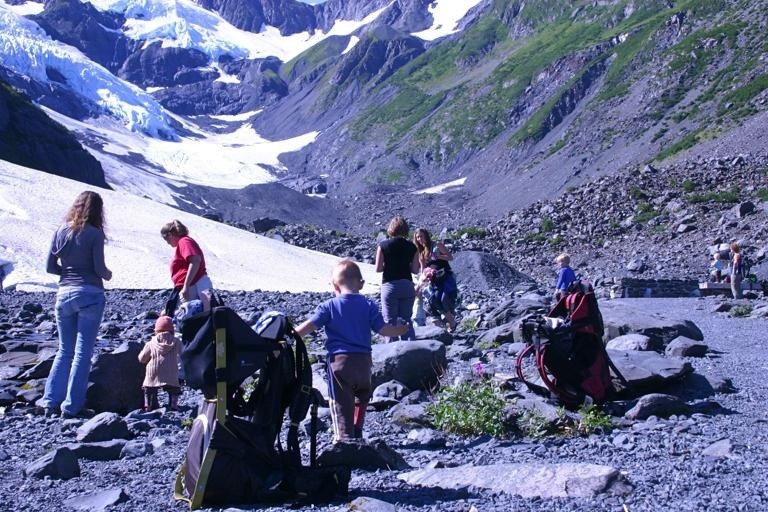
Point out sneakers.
[45,409,96,419]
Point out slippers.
[450,323,458,333]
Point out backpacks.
[730,252,751,281]
[515,281,616,410]
[174,305,329,510]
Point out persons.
[711,253,722,283]
[374,215,420,345]
[554,253,577,300]
[137,315,185,413]
[293,260,409,444]
[42,191,113,420]
[160,219,213,384]
[729,243,744,300]
[413,229,458,332]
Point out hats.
[155,316,175,334]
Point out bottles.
[430,239,441,260]
